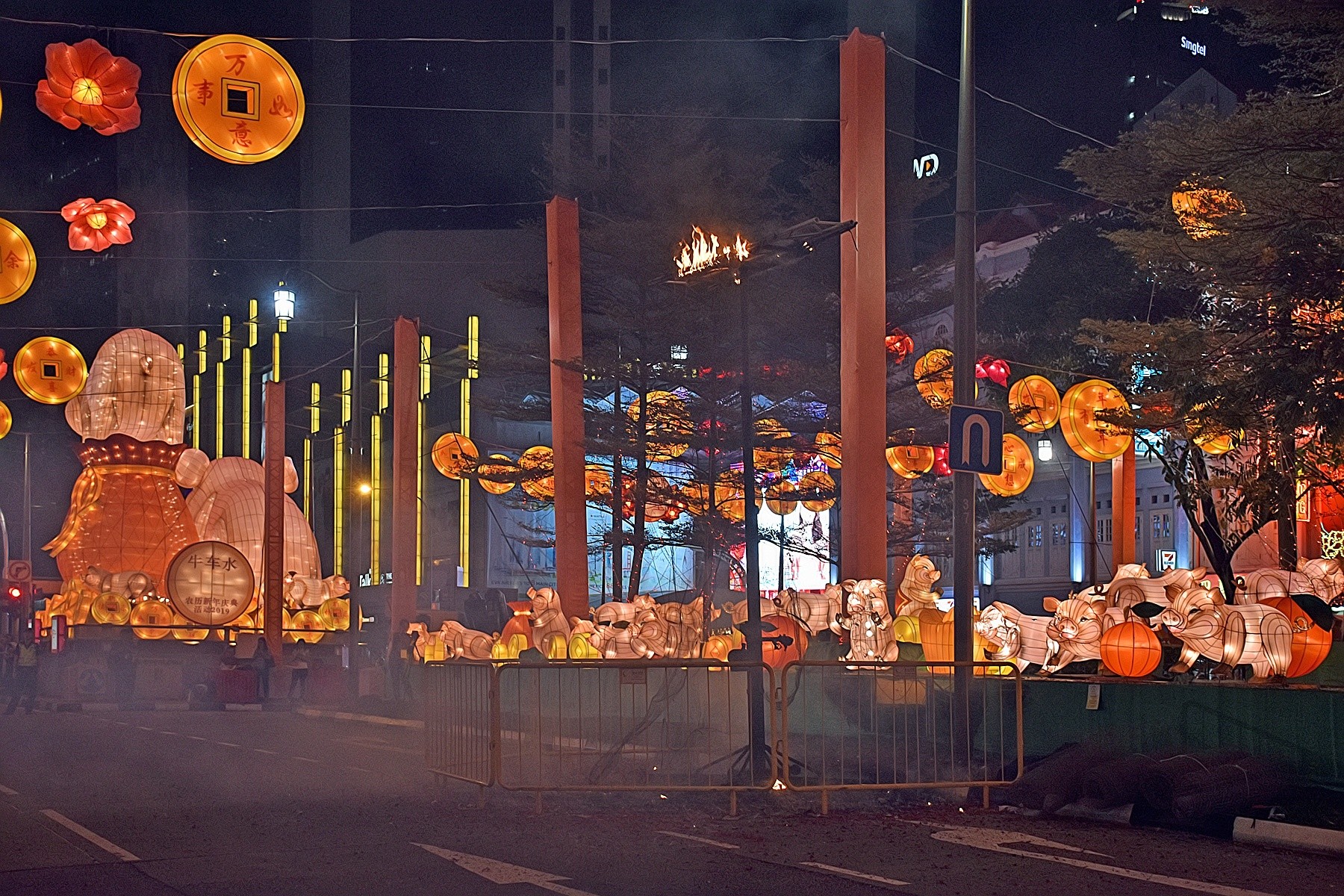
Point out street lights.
[647,213,861,788]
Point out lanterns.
[406,550,1344,683]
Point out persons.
[287,638,311,697]
[390,620,410,694]
[110,630,136,704]
[5,629,42,714]
[220,647,240,670]
[0,634,18,694]
[252,636,271,700]
